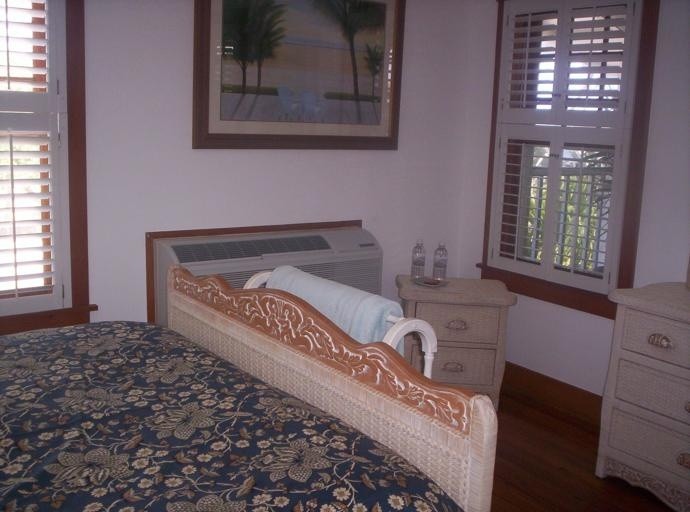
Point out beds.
[1,269,497,512]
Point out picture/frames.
[190,0,406,151]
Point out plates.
[415,279,450,287]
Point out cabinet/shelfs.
[393,273,517,418]
[590,284,689,511]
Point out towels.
[266,267,405,357]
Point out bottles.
[411,239,425,281]
[432,242,448,280]
